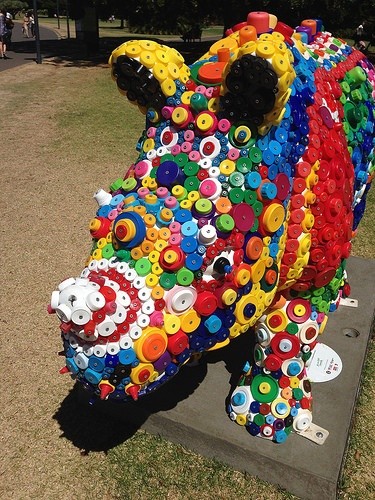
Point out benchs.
[181,29,202,43]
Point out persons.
[0,7,15,59]
[22,13,31,38]
[5,9,11,19]
[30,13,36,39]
